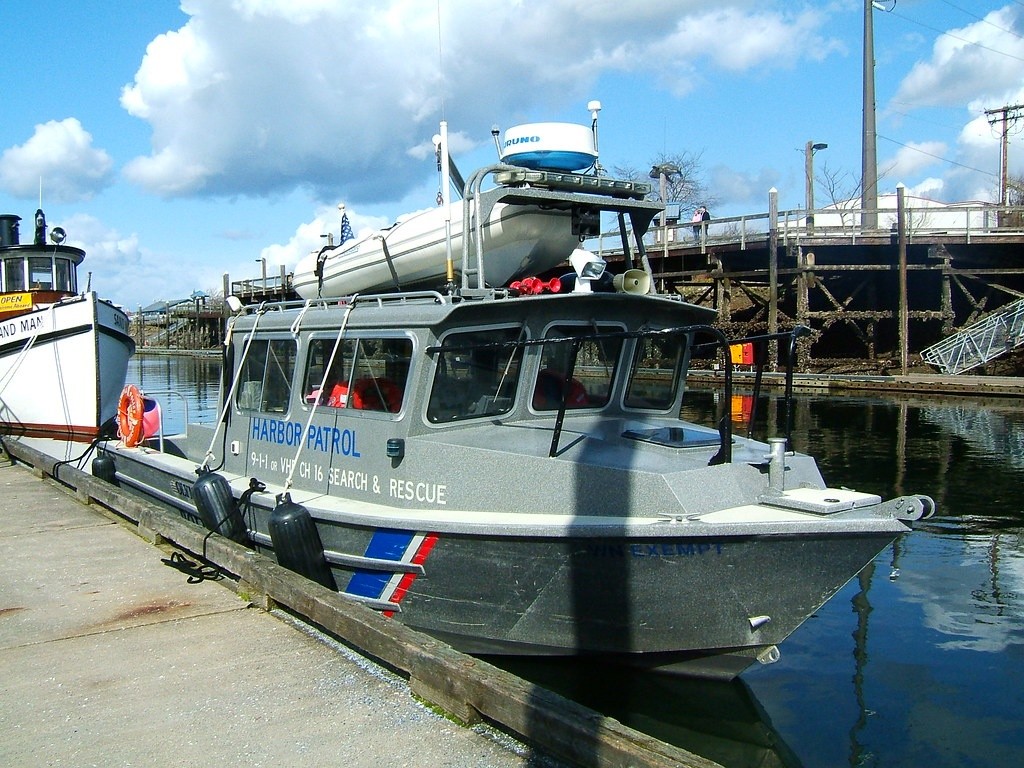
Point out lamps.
[226,296,244,311]
[570,248,607,293]
[37,214,43,227]
[50,227,67,246]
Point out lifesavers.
[117,384,145,446]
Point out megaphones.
[613,269,650,296]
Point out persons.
[692,206,710,246]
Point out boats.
[103,98,936,693]
[0,211,135,475]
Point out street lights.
[320,235,334,246]
[650,162,680,242]
[803,144,829,236]
[255,260,266,295]
